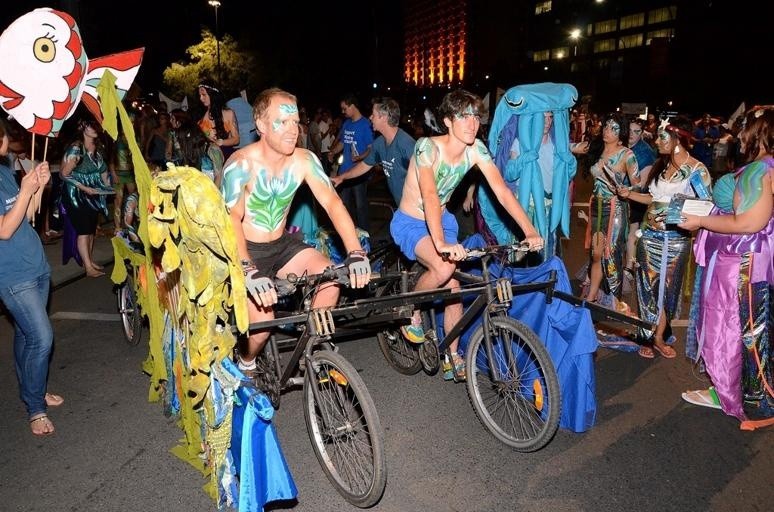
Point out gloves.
[243,264,278,295]
[333,251,371,275]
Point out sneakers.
[442,349,479,382]
[236,359,258,387]
[402,316,425,343]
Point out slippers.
[681,389,722,409]
[653,341,676,358]
[93,264,105,271]
[86,269,105,278]
[638,343,656,358]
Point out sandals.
[29,415,55,436]
[45,393,64,406]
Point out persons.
[0,112,111,438]
[109,83,542,382]
[491,105,774,429]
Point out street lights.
[206,1,225,119]
